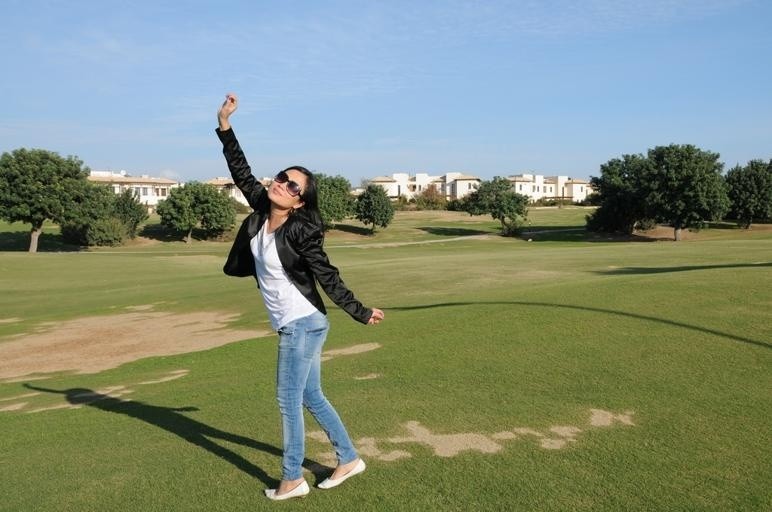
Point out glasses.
[274,172,303,200]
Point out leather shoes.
[318,459,365,489]
[265,480,310,500]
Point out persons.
[214,94,384,501]
